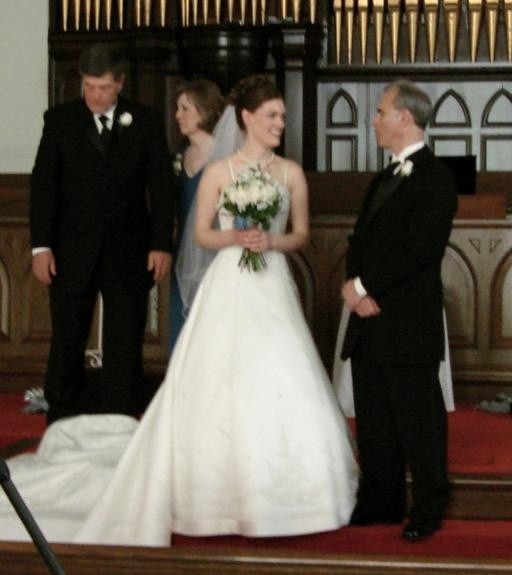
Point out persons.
[340,80,458,541]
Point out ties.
[98,116,111,145]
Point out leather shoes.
[402,512,442,540]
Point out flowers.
[217,161,285,272]
